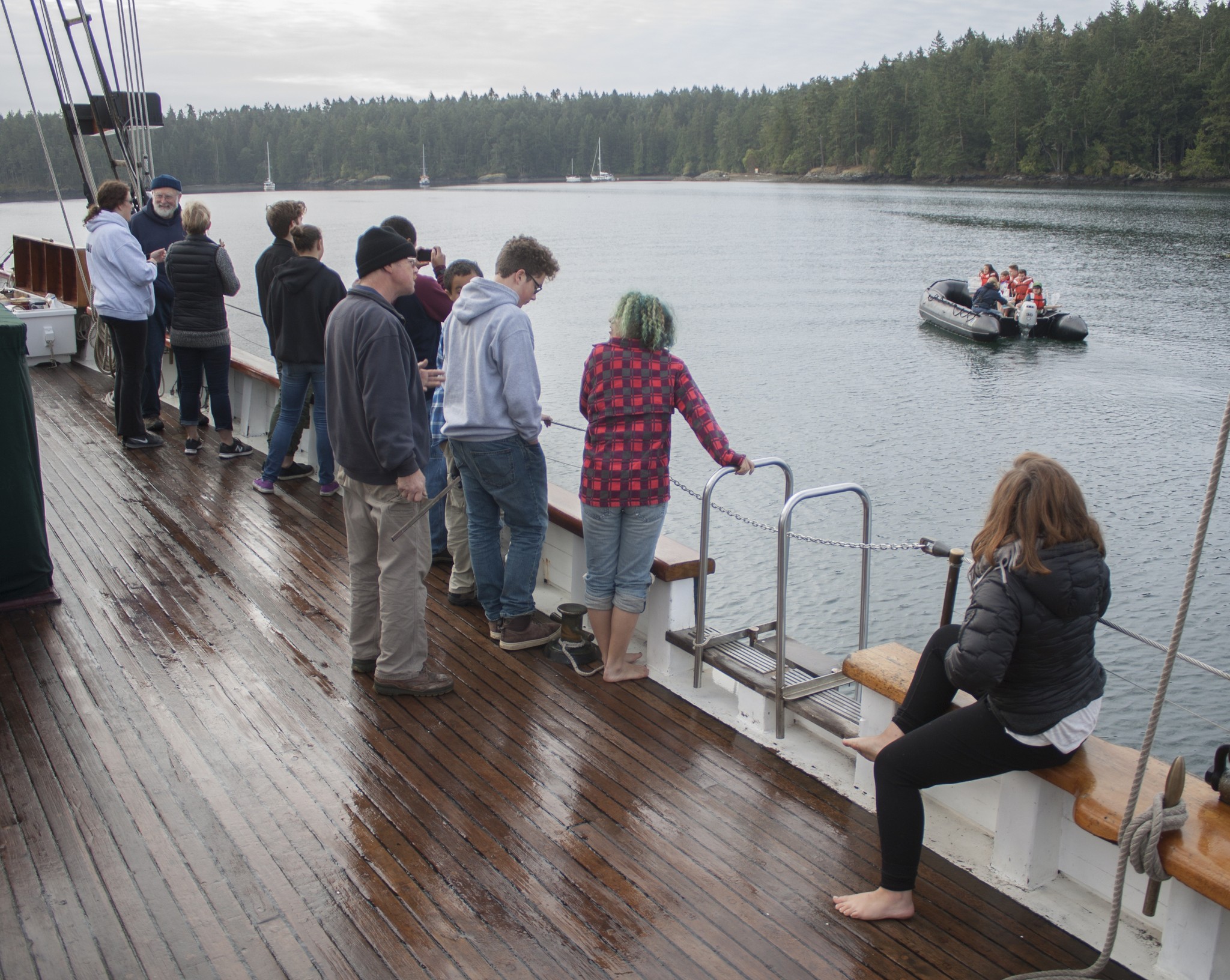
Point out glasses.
[122,197,134,205]
[405,257,416,268]
[515,268,543,295]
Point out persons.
[255,200,314,480]
[252,225,347,496]
[128,174,210,431]
[430,329,478,606]
[997,271,1011,311]
[1000,264,1034,319]
[443,259,483,303]
[351,216,453,561]
[442,235,562,651]
[83,180,165,448]
[165,201,253,458]
[833,451,1110,920]
[579,290,754,682]
[1025,281,1046,315]
[972,276,1018,315]
[324,226,454,696]
[979,264,999,287]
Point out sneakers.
[143,415,164,431]
[351,658,377,673]
[121,432,164,448]
[278,461,314,480]
[219,437,253,458]
[488,617,504,640]
[499,614,563,649]
[320,480,340,496]
[184,437,202,454]
[198,413,210,425]
[253,476,274,493]
[373,667,454,697]
[261,461,266,473]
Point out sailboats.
[264,141,275,191]
[419,144,430,184]
[590,136,615,181]
[566,158,582,180]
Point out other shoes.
[447,590,478,606]
[432,551,454,561]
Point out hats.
[1034,285,1041,289]
[356,225,417,279]
[151,174,182,192]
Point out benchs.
[542,481,715,676]
[846,642,1230,980]
[83,306,319,475]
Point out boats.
[918,279,1089,345]
